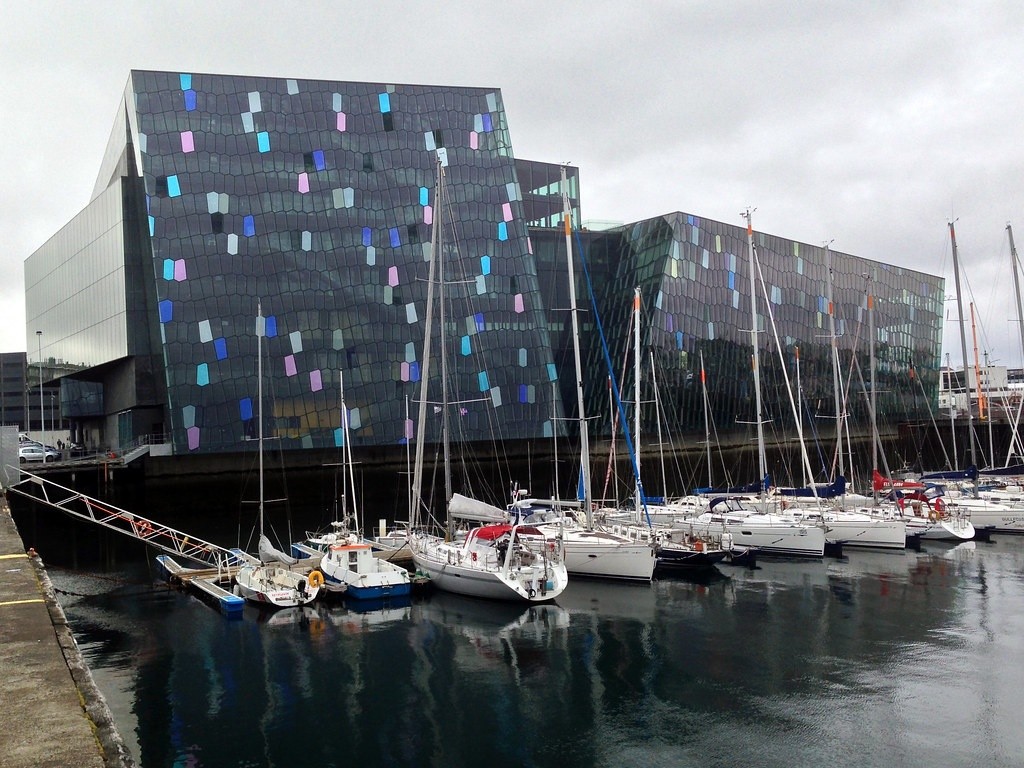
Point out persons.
[62,442,65,449]
[57,439,62,449]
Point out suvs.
[19,441,56,452]
[69,442,87,457]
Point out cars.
[19,447,58,463]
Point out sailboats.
[235,155,1024,610]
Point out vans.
[19,434,34,444]
